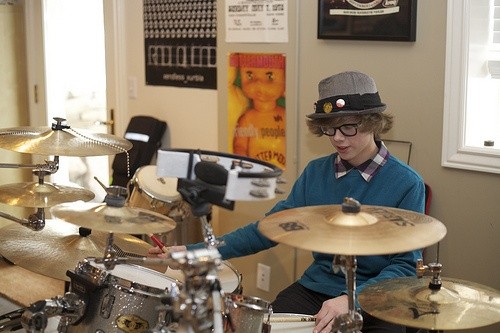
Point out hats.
[306,71,386,118]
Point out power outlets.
[256,262,272,293]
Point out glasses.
[321,121,363,137]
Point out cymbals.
[358,275,500,330]
[156,147,288,202]
[0,182,95,208]
[0,125,133,156]
[0,220,169,283]
[50,201,176,235]
[258,206,447,256]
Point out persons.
[145,71,426,333]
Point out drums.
[164,260,244,294]
[128,166,185,218]
[57,256,184,333]
[263,313,315,333]
[222,294,272,333]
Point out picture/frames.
[318,0,418,42]
